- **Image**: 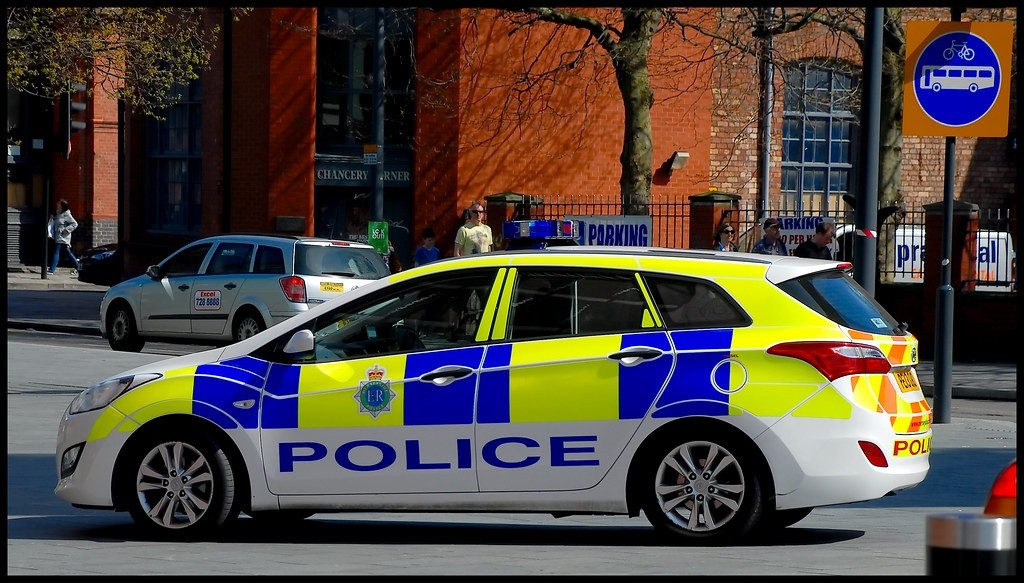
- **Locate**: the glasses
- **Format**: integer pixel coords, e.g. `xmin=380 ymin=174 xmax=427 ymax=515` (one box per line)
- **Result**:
xmin=722 ymin=230 xmax=735 ymax=234
xmin=471 ymin=210 xmax=485 ymax=214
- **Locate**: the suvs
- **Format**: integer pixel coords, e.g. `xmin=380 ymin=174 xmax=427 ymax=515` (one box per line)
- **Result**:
xmin=98 ymin=233 xmax=390 ymax=353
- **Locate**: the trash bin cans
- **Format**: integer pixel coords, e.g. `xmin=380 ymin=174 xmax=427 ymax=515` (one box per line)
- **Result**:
xmin=925 ymin=511 xmax=1016 ymax=576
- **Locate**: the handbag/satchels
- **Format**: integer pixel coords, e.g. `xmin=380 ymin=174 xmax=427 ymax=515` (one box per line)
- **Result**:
xmin=48 ymin=219 xmax=55 ymax=239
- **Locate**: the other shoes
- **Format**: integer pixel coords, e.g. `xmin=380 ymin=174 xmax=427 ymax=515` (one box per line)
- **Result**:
xmin=71 ymin=269 xmax=78 ymax=275
xmin=47 ymin=271 xmax=53 ymax=275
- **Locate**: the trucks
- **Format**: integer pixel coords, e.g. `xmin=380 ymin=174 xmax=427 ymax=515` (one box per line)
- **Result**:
xmin=827 ymin=221 xmax=1016 ymax=293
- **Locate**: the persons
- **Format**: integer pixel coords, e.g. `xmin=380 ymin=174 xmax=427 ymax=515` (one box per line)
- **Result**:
xmin=710 ymin=221 xmax=739 ymax=254
xmin=45 ymin=199 xmax=80 ymax=273
xmin=452 ymin=203 xmax=494 ymax=258
xmin=793 ymin=219 xmax=837 ymax=259
xmin=751 ymin=217 xmax=788 ymax=257
xmin=413 ymin=228 xmax=440 ymax=266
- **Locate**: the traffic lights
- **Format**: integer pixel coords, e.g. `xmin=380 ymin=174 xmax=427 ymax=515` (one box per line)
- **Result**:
xmin=54 ymin=72 xmax=86 ymax=158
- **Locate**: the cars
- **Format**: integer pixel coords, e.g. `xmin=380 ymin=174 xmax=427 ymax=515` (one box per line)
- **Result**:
xmin=78 ymin=242 xmax=118 ymax=287
xmin=50 ymin=243 xmax=932 ymax=550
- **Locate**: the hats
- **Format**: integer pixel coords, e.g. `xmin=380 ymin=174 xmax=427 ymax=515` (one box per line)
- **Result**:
xmin=763 ymin=218 xmax=779 ymax=230
